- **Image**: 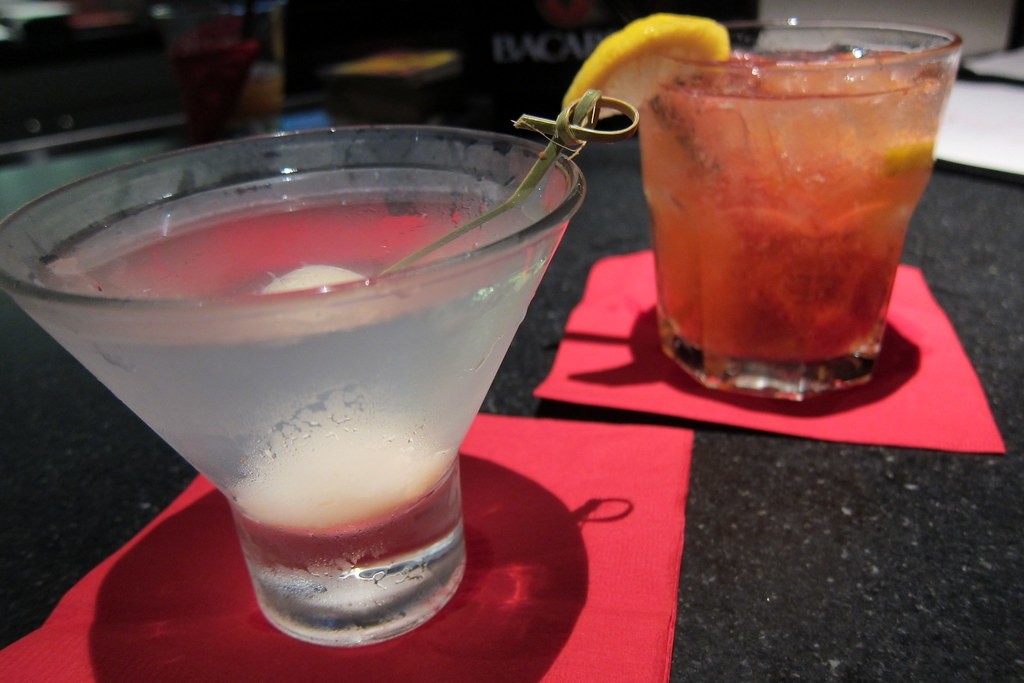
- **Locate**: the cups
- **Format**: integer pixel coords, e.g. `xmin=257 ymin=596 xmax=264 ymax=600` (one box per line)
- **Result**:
xmin=0 ymin=125 xmax=587 ymax=647
xmin=637 ymin=16 xmax=963 ymax=400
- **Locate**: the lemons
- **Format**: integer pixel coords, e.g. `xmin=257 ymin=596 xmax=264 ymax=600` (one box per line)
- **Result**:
xmin=560 ymin=14 xmax=730 ymax=120
